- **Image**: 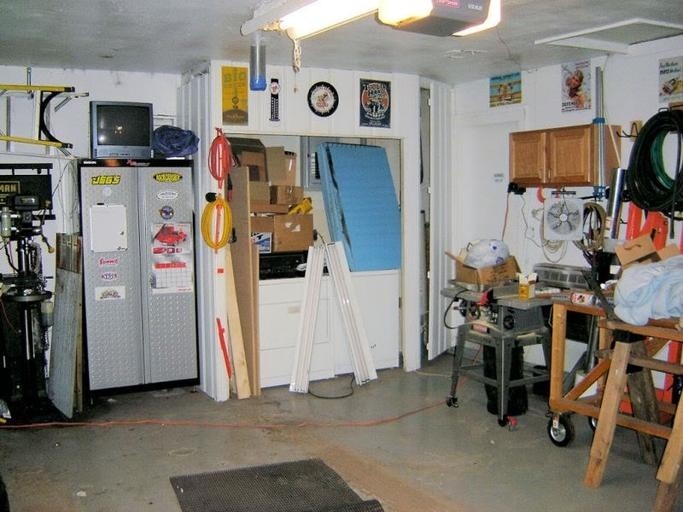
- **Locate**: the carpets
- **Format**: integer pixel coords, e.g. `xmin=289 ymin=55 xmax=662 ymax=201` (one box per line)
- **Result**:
xmin=167 ymin=455 xmax=385 ymax=512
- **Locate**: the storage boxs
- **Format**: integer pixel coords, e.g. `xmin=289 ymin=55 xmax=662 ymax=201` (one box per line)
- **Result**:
xmin=443 ymin=250 xmax=523 ymax=291
xmin=238 ymin=146 xmax=314 ymax=252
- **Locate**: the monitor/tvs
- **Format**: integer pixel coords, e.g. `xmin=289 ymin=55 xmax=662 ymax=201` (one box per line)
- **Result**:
xmin=89 ymin=101 xmax=153 ymax=160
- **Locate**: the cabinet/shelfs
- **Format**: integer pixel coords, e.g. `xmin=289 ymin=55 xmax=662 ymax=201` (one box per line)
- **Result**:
xmin=508 ymin=123 xmax=623 ymax=189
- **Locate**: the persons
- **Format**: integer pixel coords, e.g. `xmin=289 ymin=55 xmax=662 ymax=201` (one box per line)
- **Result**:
xmin=560 ymin=70 xmax=585 ymax=110
